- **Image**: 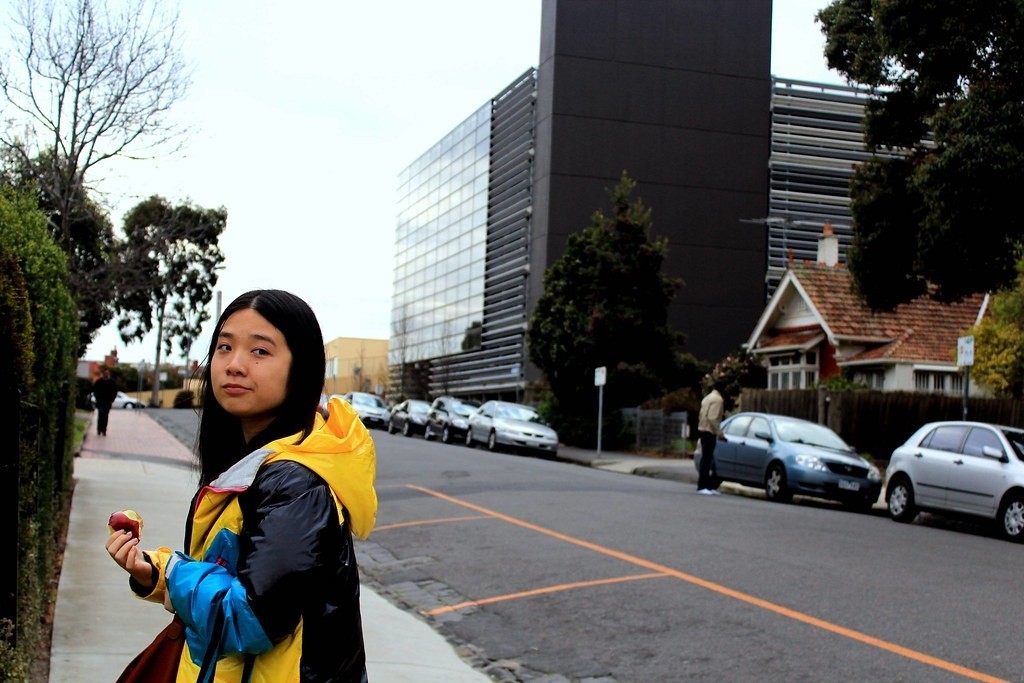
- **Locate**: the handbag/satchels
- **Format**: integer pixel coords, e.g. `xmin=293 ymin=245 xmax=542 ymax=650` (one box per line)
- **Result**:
xmin=116 ymin=613 xmax=186 ymax=683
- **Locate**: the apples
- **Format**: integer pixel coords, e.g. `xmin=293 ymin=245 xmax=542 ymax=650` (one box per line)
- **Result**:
xmin=108 ymin=509 xmax=143 ymax=544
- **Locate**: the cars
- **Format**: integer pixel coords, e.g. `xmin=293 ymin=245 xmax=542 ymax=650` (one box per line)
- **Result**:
xmin=87 ymin=390 xmax=147 ymax=411
xmin=467 ymin=400 xmax=559 ymax=459
xmin=386 ymin=399 xmax=428 ymax=437
xmin=884 ymin=422 xmax=1024 ymax=544
xmin=344 ymin=392 xmax=391 ymax=430
xmin=316 ymin=393 xmax=347 ymax=419
xmin=426 ymin=395 xmax=484 ymax=444
xmin=693 ymin=411 xmax=883 ymax=511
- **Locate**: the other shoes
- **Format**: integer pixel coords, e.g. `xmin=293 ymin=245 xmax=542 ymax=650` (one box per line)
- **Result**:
xmin=697 ymin=489 xmax=712 ymax=495
xmin=710 ymin=489 xmax=721 ymax=496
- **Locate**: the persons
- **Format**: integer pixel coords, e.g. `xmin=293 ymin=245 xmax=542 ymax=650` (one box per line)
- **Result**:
xmin=696 ymin=379 xmax=727 ymax=495
xmin=105 ymin=290 xmax=379 ymax=683
xmin=92 ymin=370 xmax=117 ymax=436
